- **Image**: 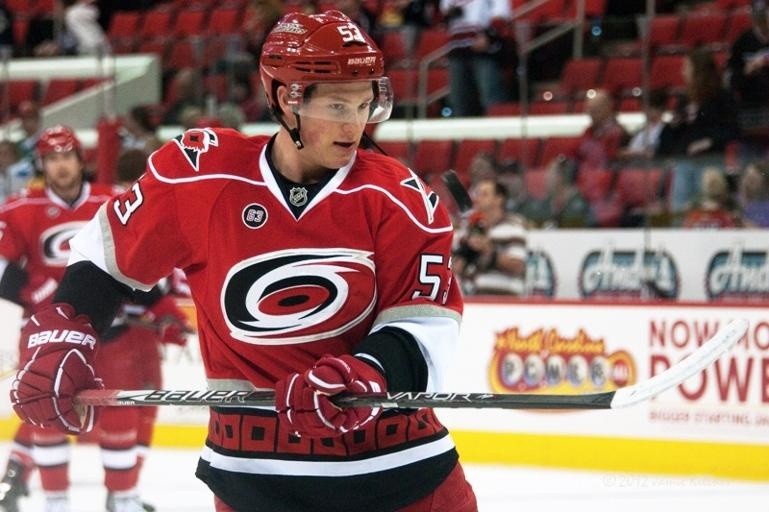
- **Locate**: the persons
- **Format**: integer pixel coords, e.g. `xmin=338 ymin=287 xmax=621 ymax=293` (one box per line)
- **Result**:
xmin=0 ymin=123 xmax=147 ymax=511
xmin=0 ymin=150 xmax=194 ymax=510
xmin=0 ymin=1 xmax=769 ymax=297
xmin=11 ymin=8 xmax=480 ymax=512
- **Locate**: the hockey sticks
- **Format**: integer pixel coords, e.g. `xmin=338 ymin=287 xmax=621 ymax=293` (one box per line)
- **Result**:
xmin=69 ymin=308 xmax=751 ymax=409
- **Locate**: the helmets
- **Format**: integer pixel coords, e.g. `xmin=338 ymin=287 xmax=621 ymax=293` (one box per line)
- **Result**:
xmin=257 ymin=8 xmax=389 ymax=113
xmin=35 ymin=125 xmax=82 ymax=160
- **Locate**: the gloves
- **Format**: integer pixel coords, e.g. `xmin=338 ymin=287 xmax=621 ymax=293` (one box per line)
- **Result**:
xmin=145 ymin=294 xmax=194 ymax=349
xmin=7 ymin=301 xmax=107 ymax=437
xmin=274 ymin=351 xmax=393 ymax=441
xmin=17 ymin=270 xmax=64 ymax=314
